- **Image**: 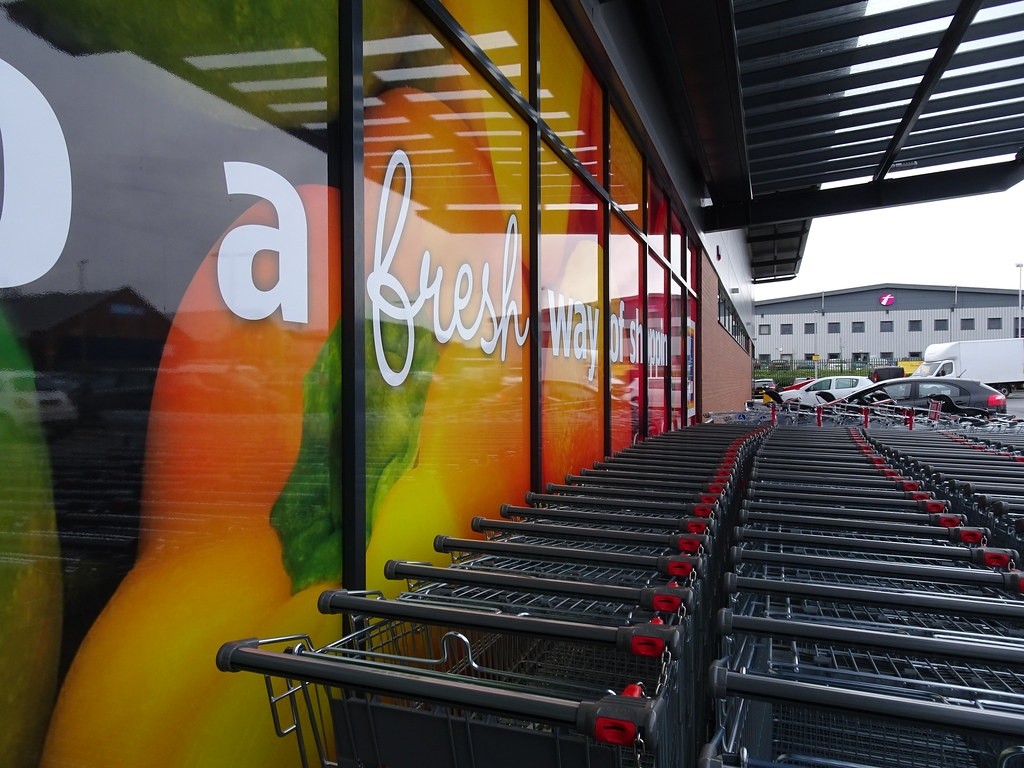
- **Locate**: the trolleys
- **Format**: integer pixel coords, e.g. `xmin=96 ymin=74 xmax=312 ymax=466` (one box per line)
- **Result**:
xmin=217 ymin=410 xmax=1024 ymax=768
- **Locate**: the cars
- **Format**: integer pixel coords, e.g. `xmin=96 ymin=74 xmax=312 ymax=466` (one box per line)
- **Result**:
xmin=0 ymin=344 xmax=698 ymax=768
xmin=813 ymin=376 xmax=1008 ymax=418
xmin=749 ymin=364 xmax=906 ymax=404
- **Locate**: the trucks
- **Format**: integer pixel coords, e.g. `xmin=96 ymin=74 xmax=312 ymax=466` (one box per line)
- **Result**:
xmin=913 ymin=337 xmax=1023 ymax=396
